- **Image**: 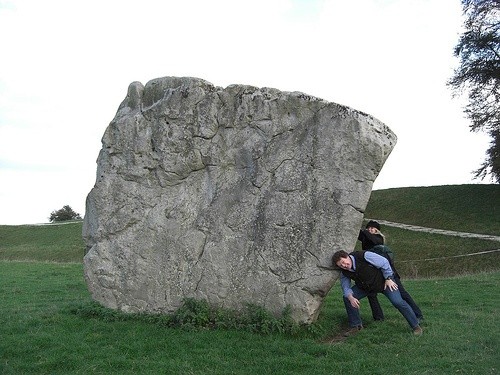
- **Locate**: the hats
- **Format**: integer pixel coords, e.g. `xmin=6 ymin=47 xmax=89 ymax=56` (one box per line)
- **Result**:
xmin=366 ymin=220 xmax=381 ymax=230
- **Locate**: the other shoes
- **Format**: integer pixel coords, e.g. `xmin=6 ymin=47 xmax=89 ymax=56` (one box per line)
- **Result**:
xmin=344 ymin=327 xmax=360 ymax=337
xmin=413 ymin=325 xmax=422 ymax=336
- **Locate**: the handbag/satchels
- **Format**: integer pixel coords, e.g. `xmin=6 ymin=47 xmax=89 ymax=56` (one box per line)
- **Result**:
xmin=368 ymin=245 xmax=394 ymax=265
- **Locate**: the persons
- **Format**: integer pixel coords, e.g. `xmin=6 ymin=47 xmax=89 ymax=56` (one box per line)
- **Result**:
xmin=332 ymin=250 xmax=422 ymax=336
xmin=355 ymin=221 xmax=423 ymax=322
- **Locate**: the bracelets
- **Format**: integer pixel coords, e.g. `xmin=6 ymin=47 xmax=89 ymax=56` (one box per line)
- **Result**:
xmin=385 ymin=276 xmax=391 ymax=280
